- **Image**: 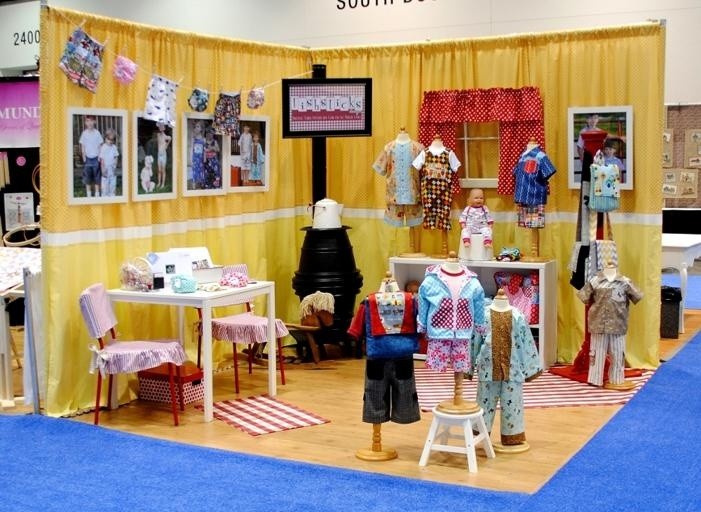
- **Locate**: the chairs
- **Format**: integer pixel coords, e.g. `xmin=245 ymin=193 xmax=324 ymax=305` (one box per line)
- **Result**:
xmin=77 ymin=283 xmax=188 ymax=427
xmin=194 ymin=264 xmax=286 ymax=395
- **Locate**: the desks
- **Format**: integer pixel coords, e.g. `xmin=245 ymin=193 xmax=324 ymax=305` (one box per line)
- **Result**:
xmin=100 ymin=274 xmax=278 ymax=426
xmin=659 ymin=233 xmax=701 ymax=335
xmin=1 ymin=246 xmax=44 ymax=411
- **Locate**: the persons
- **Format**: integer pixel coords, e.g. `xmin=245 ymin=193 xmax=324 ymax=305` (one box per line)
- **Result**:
xmin=347 ymin=271 xmax=422 ymax=423
xmin=577 ymin=115 xmax=606 ymax=183
xmin=463 ymin=289 xmax=543 ymax=443
xmin=204 ymin=129 xmax=220 ymax=188
xmin=237 ymin=125 xmax=253 ymax=184
xmin=577 ymin=268 xmax=642 ymax=388
xmin=99 ymin=128 xmax=120 ymax=196
xmin=374 ymin=128 xmax=426 ymax=229
xmin=514 ymin=137 xmax=557 ymax=229
xmin=251 ymin=132 xmax=264 ymax=185
xmin=156 ymin=125 xmax=171 ymax=191
xmin=412 ymin=136 xmax=463 ymax=232
xmin=191 ymin=121 xmax=207 ymax=189
xmin=417 ymin=251 xmax=486 ymax=374
xmin=79 ymin=115 xmax=105 ymax=197
xmin=459 ymin=188 xmax=493 ymax=247
xmin=604 ymin=141 xmax=624 ymax=183
xmin=140 ymin=155 xmax=155 ymax=193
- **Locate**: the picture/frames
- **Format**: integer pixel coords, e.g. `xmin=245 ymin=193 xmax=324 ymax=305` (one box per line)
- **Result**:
xmin=65 ymin=106 xmax=128 ymax=205
xmin=180 ymin=110 xmax=227 ymax=197
xmin=132 ymin=109 xmax=178 ymax=203
xmin=227 ymin=113 xmax=270 ymax=193
xmin=565 ymin=106 xmax=634 ymax=191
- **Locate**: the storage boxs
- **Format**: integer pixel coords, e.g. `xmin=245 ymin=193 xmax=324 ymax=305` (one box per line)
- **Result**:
xmin=134 ymin=365 xmax=205 ymax=408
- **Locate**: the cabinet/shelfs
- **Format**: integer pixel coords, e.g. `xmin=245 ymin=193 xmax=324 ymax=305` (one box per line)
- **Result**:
xmin=387 ymin=256 xmax=559 ymax=371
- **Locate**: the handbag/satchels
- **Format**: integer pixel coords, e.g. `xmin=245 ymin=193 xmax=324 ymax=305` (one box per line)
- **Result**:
xmin=588 ymin=149 xmax=620 ymax=212
xmin=584 ymin=239 xmax=618 ymax=283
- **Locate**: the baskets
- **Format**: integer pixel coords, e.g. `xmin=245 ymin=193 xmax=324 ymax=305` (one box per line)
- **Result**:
xmin=137 ymin=370 xmax=204 ymax=407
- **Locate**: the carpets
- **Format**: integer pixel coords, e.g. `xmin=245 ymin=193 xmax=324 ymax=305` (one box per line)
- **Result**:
xmin=410 ymin=366 xmax=658 ymax=412
xmin=193 ymin=393 xmax=330 ymax=437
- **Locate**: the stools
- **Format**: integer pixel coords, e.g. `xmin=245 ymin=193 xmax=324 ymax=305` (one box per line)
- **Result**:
xmin=418 ymin=406 xmax=496 ymax=473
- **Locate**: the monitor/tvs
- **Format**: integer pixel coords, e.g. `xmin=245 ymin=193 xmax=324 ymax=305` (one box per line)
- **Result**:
xmin=281 ymin=77 xmax=373 ymax=139
xmin=1 ymin=76 xmax=40 ymax=147
xmin=662 ymin=207 xmax=701 ymax=235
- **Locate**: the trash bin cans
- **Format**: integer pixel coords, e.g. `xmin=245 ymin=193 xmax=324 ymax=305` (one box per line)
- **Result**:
xmin=660 ymin=286 xmax=682 ymax=339
